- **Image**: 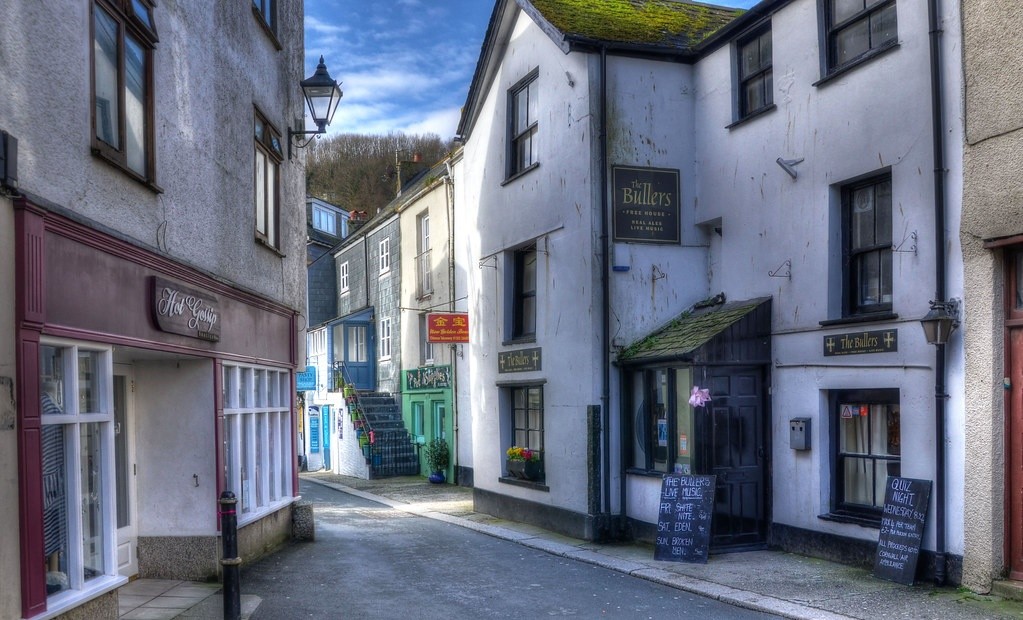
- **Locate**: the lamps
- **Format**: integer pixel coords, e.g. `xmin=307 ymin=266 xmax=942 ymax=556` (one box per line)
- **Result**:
xmin=287 ymin=53 xmax=343 ymax=160
xmin=922 ymin=298 xmax=960 ymax=345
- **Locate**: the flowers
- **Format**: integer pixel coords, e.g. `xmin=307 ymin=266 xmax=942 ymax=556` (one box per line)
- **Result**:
xmin=509 ymin=447 xmax=534 ymax=461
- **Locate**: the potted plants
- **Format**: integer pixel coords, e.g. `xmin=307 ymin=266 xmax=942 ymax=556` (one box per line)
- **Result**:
xmin=336 ymin=373 xmax=373 ymax=459
xmin=424 ymin=438 xmax=449 ymax=485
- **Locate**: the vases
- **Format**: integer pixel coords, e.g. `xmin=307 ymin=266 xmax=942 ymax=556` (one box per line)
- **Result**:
xmin=507 ymin=458 xmax=541 ymax=481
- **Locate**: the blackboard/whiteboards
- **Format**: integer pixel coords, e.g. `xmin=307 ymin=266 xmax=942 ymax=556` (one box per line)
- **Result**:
xmin=653 ymin=473 xmax=717 ymax=565
xmin=872 ymin=475 xmax=933 ymax=587
